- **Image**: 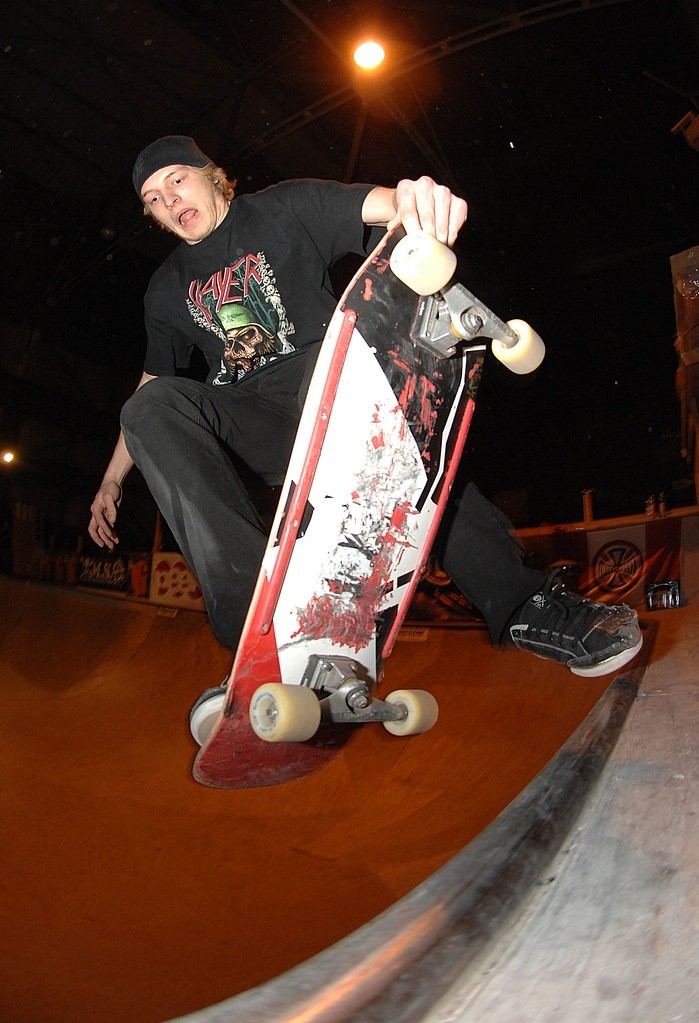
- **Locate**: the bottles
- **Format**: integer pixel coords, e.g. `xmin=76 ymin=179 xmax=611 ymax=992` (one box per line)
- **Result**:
xmin=582 ymin=489 xmax=593 ymax=521
xmin=658 ymin=492 xmax=666 ymax=518
xmin=645 ymin=495 xmax=656 ymax=521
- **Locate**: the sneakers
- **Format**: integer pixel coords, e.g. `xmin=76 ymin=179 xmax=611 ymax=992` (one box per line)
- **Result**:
xmin=190 ymin=674 xmax=229 ymax=747
xmin=506 ymin=575 xmax=643 ymax=677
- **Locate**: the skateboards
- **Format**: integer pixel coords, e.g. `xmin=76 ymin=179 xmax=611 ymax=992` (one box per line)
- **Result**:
xmin=187 ymin=217 xmax=549 ymax=794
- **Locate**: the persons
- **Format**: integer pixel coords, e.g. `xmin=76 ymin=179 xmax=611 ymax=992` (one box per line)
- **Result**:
xmin=89 ymin=138 xmax=641 ymax=672
xmin=76 ymin=528 xmax=108 ymax=555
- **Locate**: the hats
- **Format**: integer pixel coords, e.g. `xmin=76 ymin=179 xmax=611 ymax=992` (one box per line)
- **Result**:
xmin=130 ymin=135 xmax=217 ymax=200
